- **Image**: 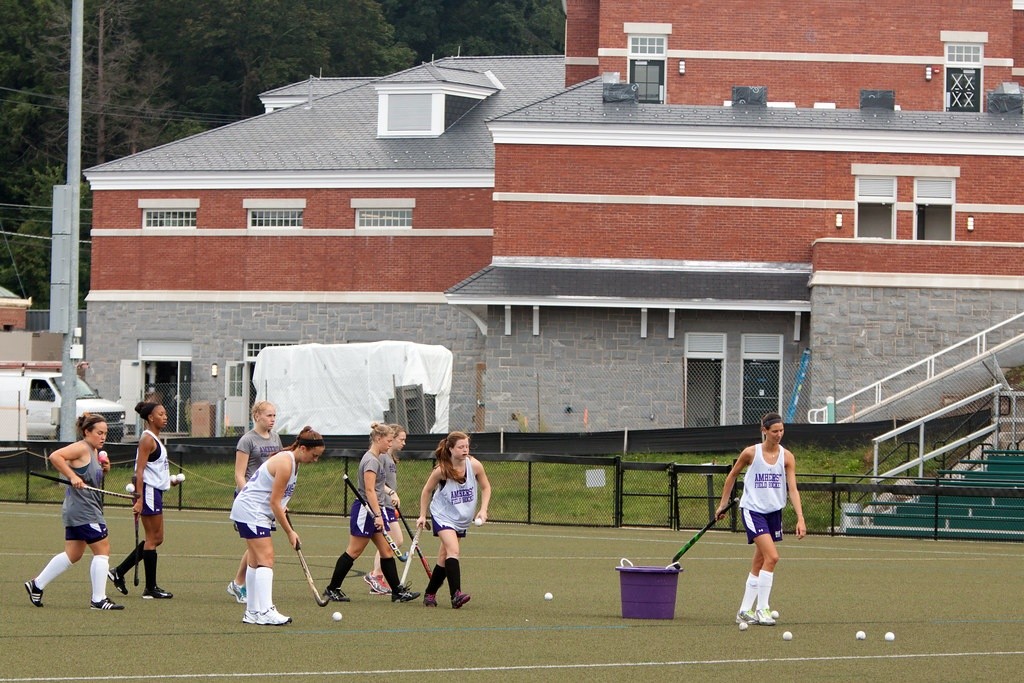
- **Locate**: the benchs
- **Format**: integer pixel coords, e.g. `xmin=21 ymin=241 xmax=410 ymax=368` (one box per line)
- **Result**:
xmin=845 ymin=450 xmax=1024 ymax=539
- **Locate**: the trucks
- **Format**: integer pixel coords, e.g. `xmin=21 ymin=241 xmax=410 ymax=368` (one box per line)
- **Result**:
xmin=0 ymin=359 xmax=127 ymax=443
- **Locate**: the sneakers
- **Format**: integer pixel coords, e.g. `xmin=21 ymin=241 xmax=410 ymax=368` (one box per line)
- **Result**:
xmin=324 ymin=586 xmax=350 ymax=603
xmin=242 ymin=610 xmax=258 ymax=623
xmin=451 ymin=589 xmax=471 ymax=608
xmin=90 ymin=597 xmax=124 ymax=611
xmin=227 ymin=581 xmax=246 ymax=603
xmin=392 ymin=585 xmax=422 ymax=602
xmin=736 ymin=608 xmax=760 ymax=625
xmin=109 ymin=567 xmax=129 ymax=596
xmin=364 ymin=572 xmax=388 ymax=594
xmin=24 ymin=580 xmax=44 ymax=608
xmin=142 ymin=586 xmax=173 ymax=600
xmin=255 ymin=607 xmax=293 ymax=626
xmin=369 ymin=583 xmax=393 ymax=595
xmin=423 ymin=594 xmax=437 ymax=607
xmin=756 ymin=607 xmax=776 ymax=625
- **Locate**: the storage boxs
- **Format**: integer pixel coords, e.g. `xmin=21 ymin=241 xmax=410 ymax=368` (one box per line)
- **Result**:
xmin=192 ymin=405 xmax=216 ymax=437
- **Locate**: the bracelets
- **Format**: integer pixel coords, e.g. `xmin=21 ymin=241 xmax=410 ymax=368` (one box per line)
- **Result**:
xmin=797 ymin=513 xmax=803 ymax=515
xmin=374 ymin=512 xmax=382 ymax=517
xmin=388 ymin=489 xmax=395 ymax=495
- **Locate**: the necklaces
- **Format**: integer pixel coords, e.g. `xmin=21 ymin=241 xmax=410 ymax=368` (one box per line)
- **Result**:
xmin=763 ymin=442 xmax=779 ymax=458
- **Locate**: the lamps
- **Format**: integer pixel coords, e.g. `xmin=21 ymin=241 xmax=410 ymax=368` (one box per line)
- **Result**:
xmin=967 ymin=215 xmax=974 ymax=233
xmin=678 ymin=59 xmax=686 ymax=76
xmin=212 ymin=362 xmax=218 ymax=377
xmin=926 ymin=65 xmax=932 ymax=82
xmin=835 ymin=212 xmax=842 ymax=229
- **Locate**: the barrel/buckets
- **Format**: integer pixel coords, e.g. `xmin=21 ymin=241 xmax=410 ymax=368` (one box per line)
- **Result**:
xmin=615 ymin=558 xmax=684 ymax=620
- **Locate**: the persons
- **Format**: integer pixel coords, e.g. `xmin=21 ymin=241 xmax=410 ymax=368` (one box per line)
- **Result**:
xmin=38 ymin=383 xmax=52 ymax=400
xmin=227 ymin=401 xmax=284 ymax=603
xmin=25 ymin=413 xmax=125 ymax=611
xmin=325 ymin=423 xmax=421 ymax=603
xmin=108 ymin=401 xmax=179 ymax=598
xmin=163 ymin=375 xmax=177 ymax=432
xmin=230 ymin=425 xmax=325 ymax=625
xmin=184 ymin=397 xmax=190 ymax=431
xmin=416 ymin=432 xmax=492 ymax=608
xmin=716 ymin=413 xmax=806 ymax=626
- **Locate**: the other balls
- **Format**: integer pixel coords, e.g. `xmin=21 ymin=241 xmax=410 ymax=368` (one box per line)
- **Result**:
xmin=771 ymin=611 xmax=779 ymax=618
xmin=545 ymin=593 xmax=553 ymax=600
xmin=783 ymin=631 xmax=792 ymax=640
xmin=885 ymin=632 xmax=895 ymax=641
xmin=126 ymin=483 xmax=135 ymax=493
xmin=475 ymin=518 xmax=482 ymax=526
xmin=170 ymin=473 xmax=186 ymax=483
xmin=856 ymin=631 xmax=866 ymax=640
xmin=739 ymin=622 xmax=748 ymax=630
xmin=332 ymin=612 xmax=342 ymax=621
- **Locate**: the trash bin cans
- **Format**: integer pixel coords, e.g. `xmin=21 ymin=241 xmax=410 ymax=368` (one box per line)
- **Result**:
xmin=839 ymin=503 xmax=863 ymax=532
xmin=191 ymin=401 xmax=215 ymax=438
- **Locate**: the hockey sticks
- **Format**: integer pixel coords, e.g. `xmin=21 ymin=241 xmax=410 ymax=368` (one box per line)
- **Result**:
xmin=131 ymin=475 xmax=140 ymax=587
xmin=671 ymin=497 xmax=740 ymax=572
xmin=29 ymin=470 xmax=141 ymax=499
xmin=342 ymin=473 xmax=409 ymax=562
xmin=398 ymin=520 xmax=431 ymax=591
xmin=285 ymin=510 xmax=331 ymax=608
xmin=395 ymin=504 xmax=432 ymax=579
